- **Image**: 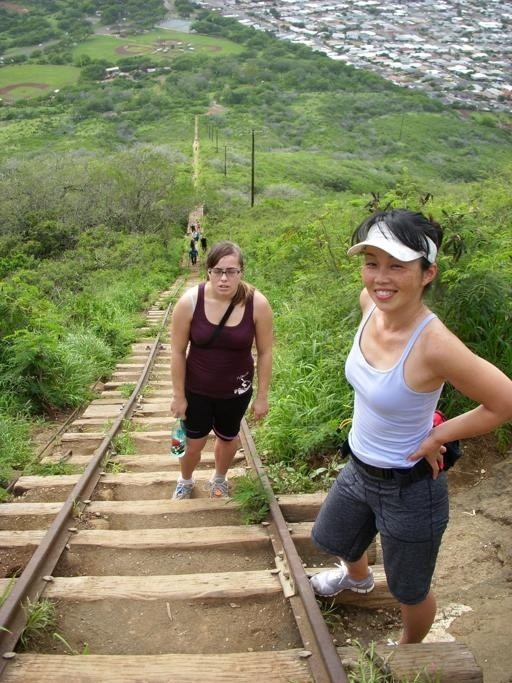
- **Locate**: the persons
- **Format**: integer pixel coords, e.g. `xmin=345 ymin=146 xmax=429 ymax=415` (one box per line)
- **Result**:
xmin=309 ymin=208 xmax=512 ymax=646
xmin=170 ymin=241 xmax=273 ymax=500
xmin=189 ymin=219 xmax=207 ymax=265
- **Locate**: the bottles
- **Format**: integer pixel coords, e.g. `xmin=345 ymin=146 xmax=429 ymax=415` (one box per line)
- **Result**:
xmin=170 ymin=417 xmax=186 ymax=458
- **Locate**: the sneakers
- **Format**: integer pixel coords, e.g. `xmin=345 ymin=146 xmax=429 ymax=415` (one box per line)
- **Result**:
xmin=172 ymin=476 xmax=195 ymax=499
xmin=210 ymin=473 xmax=228 ymax=498
xmin=310 ymin=567 xmax=374 ymax=597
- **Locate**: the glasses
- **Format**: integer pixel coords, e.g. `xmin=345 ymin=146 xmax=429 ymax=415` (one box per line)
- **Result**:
xmin=208 ymin=267 xmax=243 ymax=274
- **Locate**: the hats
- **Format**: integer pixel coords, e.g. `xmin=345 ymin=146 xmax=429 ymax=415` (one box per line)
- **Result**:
xmin=346 ymin=221 xmax=438 ymax=265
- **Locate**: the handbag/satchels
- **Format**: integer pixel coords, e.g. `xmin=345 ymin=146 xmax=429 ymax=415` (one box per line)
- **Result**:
xmin=433 ymin=410 xmax=461 ymax=472
